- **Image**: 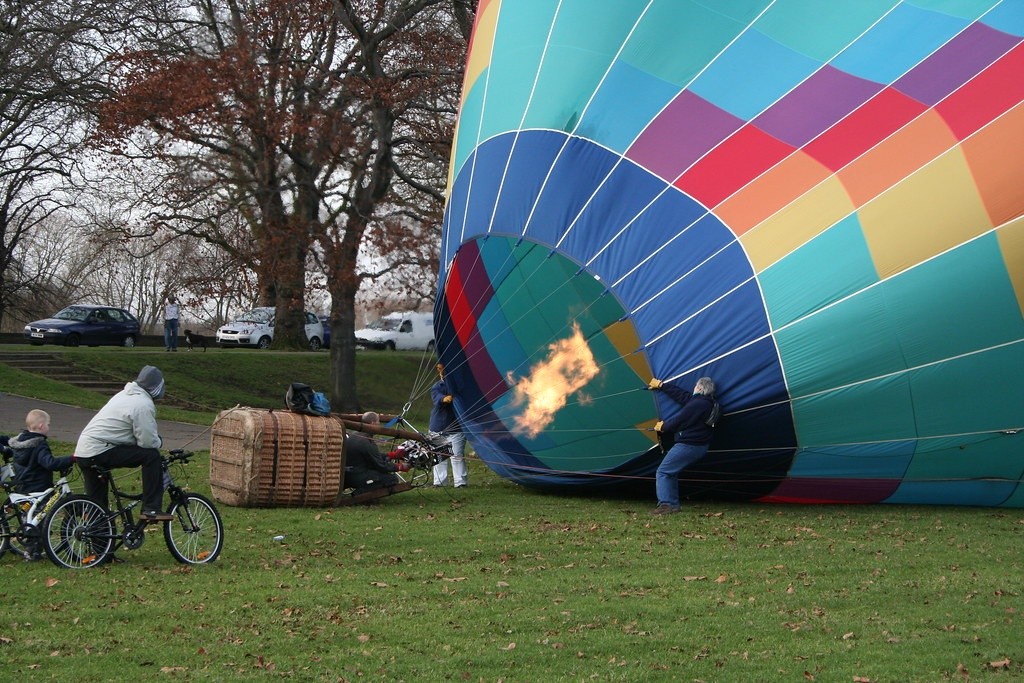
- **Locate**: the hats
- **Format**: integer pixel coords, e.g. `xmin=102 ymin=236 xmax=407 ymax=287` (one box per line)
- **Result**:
xmin=436 ymin=363 xmax=445 ymax=370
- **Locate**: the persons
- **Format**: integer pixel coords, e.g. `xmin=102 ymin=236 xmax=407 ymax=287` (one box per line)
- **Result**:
xmin=74 ymin=365 xmax=174 ymax=566
xmin=161 ymin=294 xmax=180 ymax=352
xmin=647 ymin=377 xmax=720 ymax=515
xmin=428 ymin=363 xmax=470 ymax=488
xmin=7 ymin=409 xmax=77 ymax=561
xmin=343 ymin=411 xmax=411 ymax=506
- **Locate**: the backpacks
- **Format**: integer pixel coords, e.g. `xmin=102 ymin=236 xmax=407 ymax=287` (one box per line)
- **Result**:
xmin=699 ymin=394 xmax=721 ymax=428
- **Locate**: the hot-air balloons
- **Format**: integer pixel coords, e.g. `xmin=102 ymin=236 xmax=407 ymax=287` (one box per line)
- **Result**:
xmin=210 ymin=0 xmax=1024 ymax=517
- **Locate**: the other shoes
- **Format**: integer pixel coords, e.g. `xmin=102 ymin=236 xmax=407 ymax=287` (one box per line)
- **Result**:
xmin=672 ymin=507 xmax=682 ymax=513
xmin=430 ymin=484 xmax=446 ymax=489
xmin=171 ymin=347 xmax=177 ymax=352
xmin=32 ymin=553 xmax=44 ymax=561
xmin=166 ymin=349 xmax=170 ymax=352
xmin=458 ymin=485 xmax=467 ymax=489
xmin=23 ymin=551 xmax=32 ymax=561
xmin=655 ymin=505 xmax=672 ymax=515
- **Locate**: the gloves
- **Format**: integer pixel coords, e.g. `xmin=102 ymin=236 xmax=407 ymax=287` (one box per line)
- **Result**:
xmin=387 ymin=448 xmax=406 ymax=459
xmin=654 ymin=420 xmax=664 ymax=432
xmin=443 ymin=395 xmax=452 ymax=403
xmin=394 ymin=462 xmax=412 ymax=472
xmin=648 ymin=378 xmax=662 ymax=390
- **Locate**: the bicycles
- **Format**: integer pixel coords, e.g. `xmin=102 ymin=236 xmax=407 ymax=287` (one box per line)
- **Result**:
xmin=43 ymin=451 xmax=225 ymax=570
xmin=0 ymin=449 xmax=98 ymax=565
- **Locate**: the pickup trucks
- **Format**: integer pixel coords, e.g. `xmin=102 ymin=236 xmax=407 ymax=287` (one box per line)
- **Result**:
xmin=354 ymin=311 xmax=436 ymax=352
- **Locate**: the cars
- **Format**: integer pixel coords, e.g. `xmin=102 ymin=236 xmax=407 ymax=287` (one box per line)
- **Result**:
xmin=24 ymin=305 xmax=140 ymax=348
xmin=216 ymin=307 xmax=324 ymax=351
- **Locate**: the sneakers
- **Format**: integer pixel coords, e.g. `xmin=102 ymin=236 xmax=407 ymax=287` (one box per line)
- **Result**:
xmin=140 ymin=509 xmax=173 ymax=521
